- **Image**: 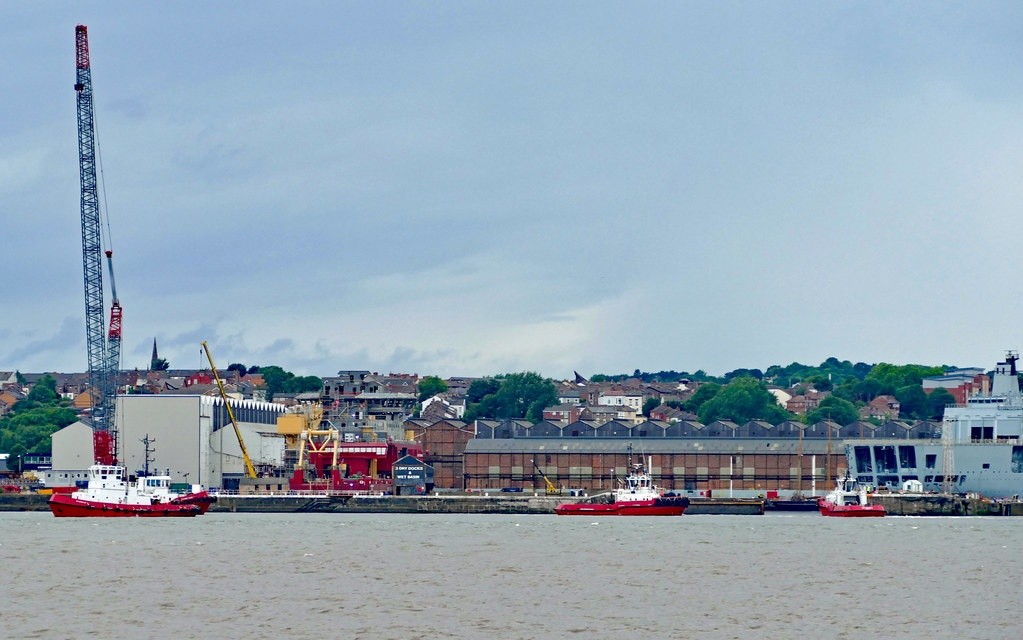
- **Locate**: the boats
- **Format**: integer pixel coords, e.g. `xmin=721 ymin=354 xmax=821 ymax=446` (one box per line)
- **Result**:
xmin=46 ymin=463 xmax=218 ymax=518
xmin=553 ymin=455 xmax=690 ymax=516
xmin=817 ymin=470 xmax=888 ymax=517
xmin=841 ymin=348 xmax=1023 ymax=504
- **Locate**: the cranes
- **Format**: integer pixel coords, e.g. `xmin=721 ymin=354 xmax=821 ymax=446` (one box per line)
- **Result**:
xmin=71 ymin=24 xmax=125 ymax=468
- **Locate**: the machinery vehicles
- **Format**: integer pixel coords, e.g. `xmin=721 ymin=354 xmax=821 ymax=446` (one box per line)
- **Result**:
xmin=199 ymin=341 xmax=276 ymax=478
xmin=530 ymin=458 xmax=557 ymax=495
